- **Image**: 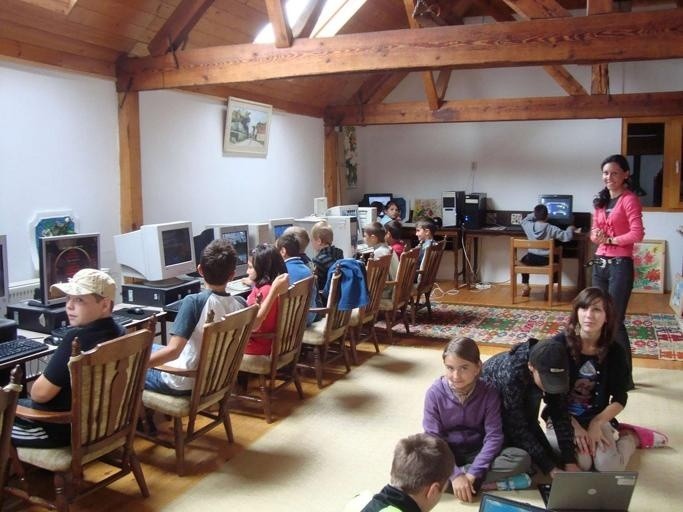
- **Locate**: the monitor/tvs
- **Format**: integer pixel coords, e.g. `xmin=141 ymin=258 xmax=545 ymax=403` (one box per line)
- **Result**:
xmin=38 ymin=233 xmax=100 ymax=306
xmin=368 ymin=194 xmax=389 ymax=216
xmin=197 ymin=224 xmax=249 ymax=280
xmin=540 ymin=195 xmax=572 ymax=225
xmin=115 ymin=222 xmax=196 ymax=287
xmin=271 ymin=217 xmax=298 ymax=244
xmin=335 ymin=204 xmax=358 ymax=216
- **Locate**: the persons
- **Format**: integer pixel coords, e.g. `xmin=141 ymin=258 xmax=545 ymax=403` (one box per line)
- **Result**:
xmin=346 ymin=428 xmax=456 ymax=512
xmin=419 ymin=335 xmax=534 ymax=504
xmin=133 ymin=238 xmax=250 ymax=436
xmin=481 ymin=335 xmax=584 ymax=481
xmin=518 ymin=202 xmax=577 ymax=302
xmin=583 ymin=152 xmax=646 ymax=393
xmin=238 ymin=197 xmax=441 ymax=357
xmin=0 ymin=266 xmax=133 ymax=451
xmin=548 ymin=287 xmax=670 ymax=474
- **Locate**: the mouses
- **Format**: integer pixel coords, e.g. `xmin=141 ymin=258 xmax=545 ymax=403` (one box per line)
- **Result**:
xmin=46 ymin=333 xmax=66 ymax=346
xmin=129 ymin=306 xmax=145 ymax=315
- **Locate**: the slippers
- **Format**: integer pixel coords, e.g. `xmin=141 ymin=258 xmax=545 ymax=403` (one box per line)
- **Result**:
xmin=617 ymin=423 xmax=669 ymax=449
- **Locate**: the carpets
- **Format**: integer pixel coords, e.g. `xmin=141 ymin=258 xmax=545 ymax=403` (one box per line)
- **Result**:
xmin=157 ymin=345 xmax=683 ymax=512
xmin=354 ymin=300 xmax=683 ymax=362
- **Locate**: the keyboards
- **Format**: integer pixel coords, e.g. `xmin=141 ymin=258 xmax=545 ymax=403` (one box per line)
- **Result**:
xmin=1 ymin=337 xmax=48 ymax=362
xmin=54 ymin=316 xmax=131 ymax=337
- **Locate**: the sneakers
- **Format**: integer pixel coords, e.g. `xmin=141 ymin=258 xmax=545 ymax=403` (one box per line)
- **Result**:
xmin=495 ymin=472 xmax=533 ymax=490
xmin=544 ymin=284 xmax=549 ymax=301
xmin=521 ymin=283 xmax=531 ymax=297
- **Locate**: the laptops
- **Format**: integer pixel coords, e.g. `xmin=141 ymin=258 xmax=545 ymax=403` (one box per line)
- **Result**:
xmin=538 ymin=471 xmax=638 ymax=511
xmin=479 ymin=491 xmax=540 ymax=511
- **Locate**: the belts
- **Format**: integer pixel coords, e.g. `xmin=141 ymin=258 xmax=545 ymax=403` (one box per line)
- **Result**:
xmin=583 ymin=256 xmax=634 ymax=268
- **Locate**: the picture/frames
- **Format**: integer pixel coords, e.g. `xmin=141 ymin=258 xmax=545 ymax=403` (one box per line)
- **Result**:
xmin=668 ymin=273 xmax=683 ymax=318
xmin=222 ymin=96 xmax=273 ymax=158
xmin=632 ymin=238 xmax=665 ymax=294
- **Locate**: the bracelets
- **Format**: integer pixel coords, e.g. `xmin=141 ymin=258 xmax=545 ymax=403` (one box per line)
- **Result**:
xmin=608 ymin=237 xmax=612 ymax=245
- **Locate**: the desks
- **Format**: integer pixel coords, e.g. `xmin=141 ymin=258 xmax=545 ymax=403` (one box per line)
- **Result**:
xmin=359 ymin=226 xmax=587 ymax=294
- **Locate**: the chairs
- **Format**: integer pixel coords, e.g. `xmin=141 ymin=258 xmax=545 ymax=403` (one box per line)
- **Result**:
xmin=510 ymin=235 xmax=563 ymax=307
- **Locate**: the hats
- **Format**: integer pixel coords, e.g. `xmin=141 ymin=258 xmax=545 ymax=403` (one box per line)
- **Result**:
xmin=528 ymin=338 xmax=570 ymax=394
xmin=49 ymin=268 xmax=117 ymax=302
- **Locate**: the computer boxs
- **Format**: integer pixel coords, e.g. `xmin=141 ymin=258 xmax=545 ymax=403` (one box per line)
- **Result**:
xmin=464 ymin=194 xmax=487 ymax=231
xmin=327 ymin=215 xmax=357 ymax=257
xmin=442 ymin=191 xmax=463 ymax=231
xmin=250 ymin=224 xmax=271 ymax=248
xmin=359 ymin=208 xmax=376 ymax=243
xmin=5 ymin=298 xmax=70 ymax=335
xmin=121 ymin=280 xmax=201 ymax=305
